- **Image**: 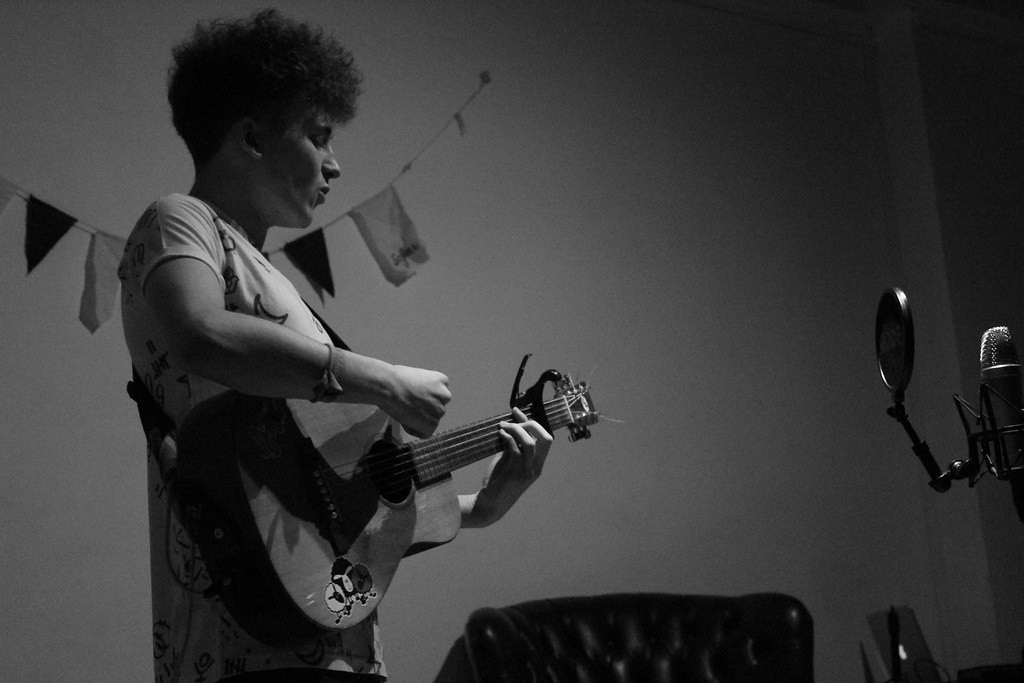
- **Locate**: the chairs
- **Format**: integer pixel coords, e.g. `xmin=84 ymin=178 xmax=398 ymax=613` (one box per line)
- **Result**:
xmin=464 ymin=591 xmax=815 ymax=683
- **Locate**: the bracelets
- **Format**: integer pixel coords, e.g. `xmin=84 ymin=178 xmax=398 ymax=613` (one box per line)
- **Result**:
xmin=309 ymin=343 xmax=346 ymax=404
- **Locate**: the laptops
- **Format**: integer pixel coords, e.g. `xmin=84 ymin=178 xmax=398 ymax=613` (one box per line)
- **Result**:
xmin=867 ymin=605 xmax=943 ymax=683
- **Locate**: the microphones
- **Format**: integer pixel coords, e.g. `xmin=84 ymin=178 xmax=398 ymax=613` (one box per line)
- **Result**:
xmin=979 ymin=326 xmax=1024 ymax=522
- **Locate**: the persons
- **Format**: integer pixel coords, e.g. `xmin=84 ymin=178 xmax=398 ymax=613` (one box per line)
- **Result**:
xmin=116 ymin=7 xmax=553 ymax=683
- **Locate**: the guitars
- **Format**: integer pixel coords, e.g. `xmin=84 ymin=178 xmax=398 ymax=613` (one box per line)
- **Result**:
xmin=179 ymin=351 xmax=599 ymax=642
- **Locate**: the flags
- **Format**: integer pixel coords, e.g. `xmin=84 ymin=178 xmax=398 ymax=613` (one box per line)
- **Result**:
xmin=349 ymin=186 xmax=432 ymax=286
